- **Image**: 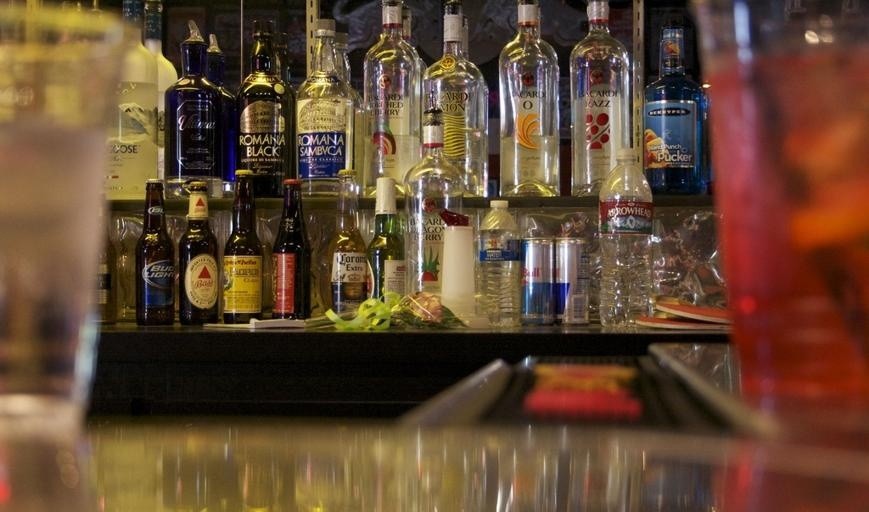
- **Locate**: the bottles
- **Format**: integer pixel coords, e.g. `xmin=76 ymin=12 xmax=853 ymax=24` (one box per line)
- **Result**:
xmin=221 ymin=169 xmax=264 ymax=323
xmin=498 ymin=0 xmax=560 ymax=196
xmin=441 ymin=226 xmax=476 ymax=322
xmin=165 ymin=18 xmax=226 ymax=175
xmin=203 ymin=33 xmax=236 ymax=175
xmin=598 ymin=147 xmax=656 ymax=327
xmin=272 ymin=31 xmax=292 ymax=85
xmin=178 ymin=180 xmax=220 ymax=324
xmin=104 ymin=0 xmax=158 ymax=198
xmin=144 ymin=0 xmax=178 ymax=181
xmin=326 ymin=170 xmax=369 ymax=313
xmin=642 ymin=20 xmax=702 ymax=190
xmin=363 ymin=0 xmax=425 ymax=185
xmin=368 ymin=176 xmax=406 ymax=306
xmin=297 ymin=19 xmax=363 ymax=195
xmin=335 ymin=32 xmax=352 ymax=86
xmin=418 ymin=0 xmax=490 ymax=201
xmin=477 ymin=200 xmax=520 ymax=327
xmin=270 ymin=179 xmax=312 ymax=320
xmin=236 ymin=19 xmax=293 ymax=181
xmin=95 ymin=191 xmax=114 ymax=328
xmin=135 ymin=179 xmax=176 ymax=326
xmin=569 ymin=0 xmax=631 ymax=197
xmin=403 ymin=107 xmax=466 ymax=302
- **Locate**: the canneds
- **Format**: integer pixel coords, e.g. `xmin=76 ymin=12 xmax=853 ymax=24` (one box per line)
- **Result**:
xmin=522 ymin=237 xmax=555 ymax=325
xmin=558 ymin=238 xmax=589 ymax=327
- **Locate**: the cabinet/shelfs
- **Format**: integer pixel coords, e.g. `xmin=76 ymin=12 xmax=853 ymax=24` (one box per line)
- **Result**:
xmin=79 ymin=0 xmax=731 ymax=437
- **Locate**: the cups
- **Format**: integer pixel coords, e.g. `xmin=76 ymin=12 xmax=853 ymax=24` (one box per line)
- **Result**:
xmin=691 ymin=0 xmax=869 ymax=448
xmin=0 ymin=7 xmax=147 ymax=449
